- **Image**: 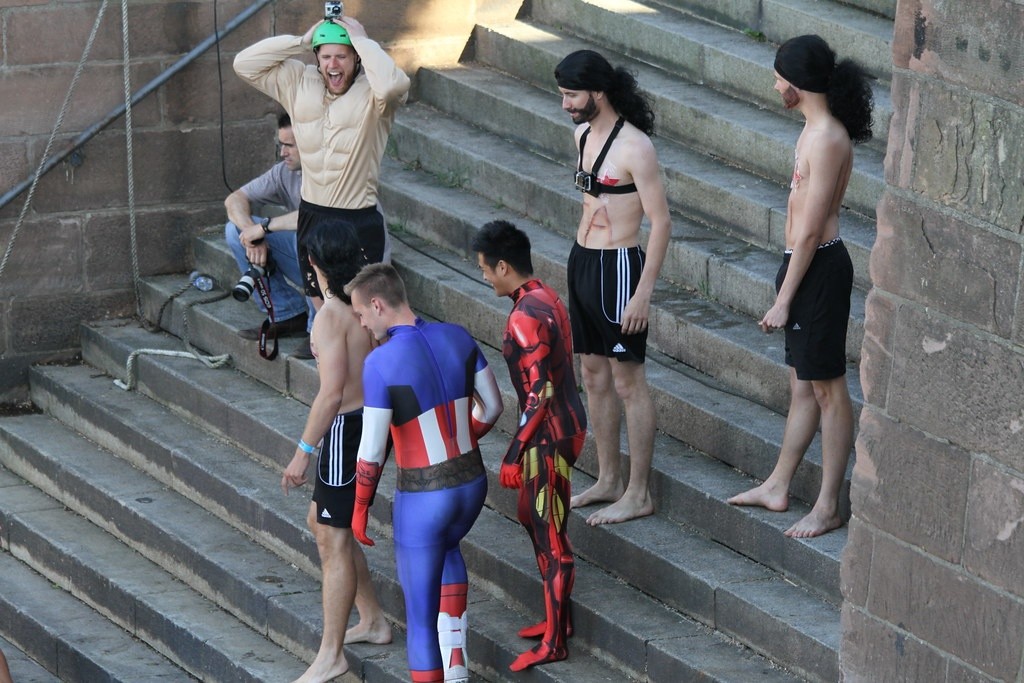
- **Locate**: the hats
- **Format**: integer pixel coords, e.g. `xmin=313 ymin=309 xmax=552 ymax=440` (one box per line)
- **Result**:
xmin=774 ymin=35 xmax=835 ymax=93
xmin=553 ymin=50 xmax=614 ymax=92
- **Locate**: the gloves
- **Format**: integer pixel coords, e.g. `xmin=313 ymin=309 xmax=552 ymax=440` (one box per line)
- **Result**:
xmin=499 ymin=462 xmax=524 ymax=490
xmin=352 ymin=507 xmax=375 ymax=547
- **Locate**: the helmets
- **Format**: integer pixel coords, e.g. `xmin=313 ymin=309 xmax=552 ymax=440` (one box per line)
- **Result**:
xmin=310 ymin=19 xmax=353 ymax=51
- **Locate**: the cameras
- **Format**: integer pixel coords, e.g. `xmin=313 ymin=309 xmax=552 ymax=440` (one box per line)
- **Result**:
xmin=325 ymin=1 xmax=343 ymax=18
xmin=231 ymin=248 xmax=277 ymax=301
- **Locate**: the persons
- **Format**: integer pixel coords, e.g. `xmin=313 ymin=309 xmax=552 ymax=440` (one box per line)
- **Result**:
xmin=472 ymin=221 xmax=588 ymax=672
xmin=232 ymin=14 xmax=409 ymax=313
xmin=726 ymin=35 xmax=876 ymax=538
xmin=282 ymin=219 xmax=390 ymax=683
xmin=556 ymin=49 xmax=672 ymax=525
xmin=224 ymin=115 xmax=392 ymax=359
xmin=343 ymin=262 xmax=504 ymax=683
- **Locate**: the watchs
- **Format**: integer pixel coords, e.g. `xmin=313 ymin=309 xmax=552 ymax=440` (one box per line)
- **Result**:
xmin=262 ymin=216 xmax=272 ymax=233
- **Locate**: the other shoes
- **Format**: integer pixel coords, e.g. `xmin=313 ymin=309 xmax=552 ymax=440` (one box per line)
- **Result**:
xmin=292 ymin=333 xmax=315 ymax=359
xmin=237 ymin=312 xmax=309 ymax=341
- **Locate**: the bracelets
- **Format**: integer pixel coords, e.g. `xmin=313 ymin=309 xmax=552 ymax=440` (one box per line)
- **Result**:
xmin=299 ymin=441 xmax=315 ymax=454
